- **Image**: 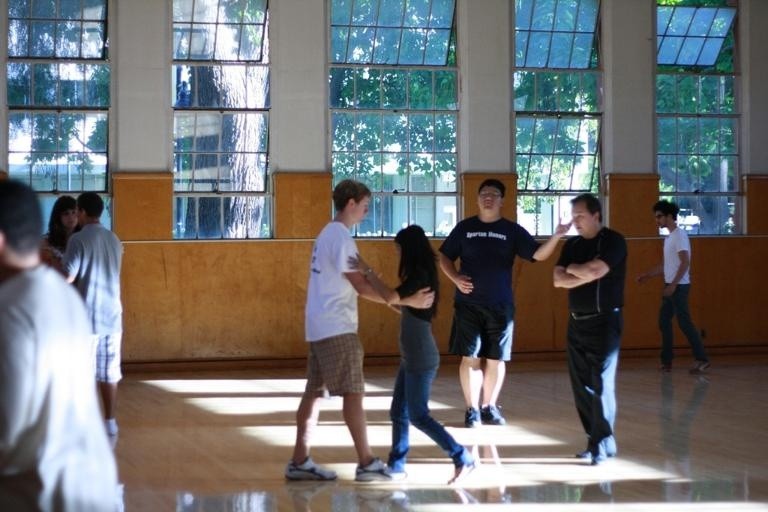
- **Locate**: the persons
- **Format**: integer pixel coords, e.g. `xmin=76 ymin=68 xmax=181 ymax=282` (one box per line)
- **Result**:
xmin=551 ymin=191 xmax=629 ymax=467
xmin=634 ymin=198 xmax=714 ymax=376
xmin=282 ymin=178 xmax=435 ymax=482
xmin=43 ymin=196 xmax=78 ymax=261
xmin=437 ymin=177 xmax=572 ymax=429
xmin=657 ymin=373 xmax=713 ymax=504
xmin=43 ymin=192 xmax=125 ymax=436
xmin=344 ymin=223 xmax=477 ymax=486
xmin=0 ymin=176 xmax=124 ymax=510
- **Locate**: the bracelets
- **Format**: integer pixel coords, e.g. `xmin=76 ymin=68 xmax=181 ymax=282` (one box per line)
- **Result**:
xmin=364 ymin=268 xmax=373 ymax=277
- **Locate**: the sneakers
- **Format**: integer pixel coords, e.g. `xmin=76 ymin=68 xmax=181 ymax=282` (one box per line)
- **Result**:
xmin=285 ymin=456 xmax=337 ymax=480
xmin=355 ymin=458 xmax=406 ymax=480
xmin=577 ymin=437 xmax=615 ymax=464
xmin=465 ymin=405 xmax=505 ymax=427
xmin=658 ymin=359 xmax=713 ymax=374
xmin=105 ymin=420 xmax=119 ymax=435
